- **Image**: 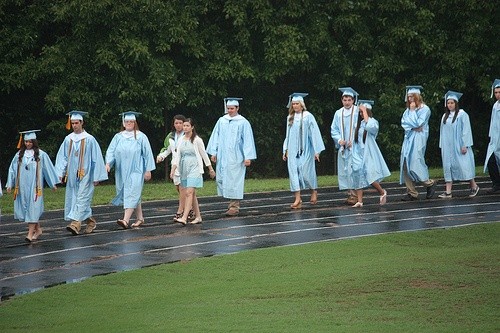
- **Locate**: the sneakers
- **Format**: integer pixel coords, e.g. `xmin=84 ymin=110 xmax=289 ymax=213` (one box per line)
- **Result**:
xmin=84 ymin=224 xmax=97 ymax=233
xmin=468 ymin=185 xmax=479 ymax=197
xmin=438 ymin=192 xmax=452 ymax=198
xmin=66 ymin=225 xmax=79 ymax=235
xmin=224 ymin=210 xmax=240 ymax=216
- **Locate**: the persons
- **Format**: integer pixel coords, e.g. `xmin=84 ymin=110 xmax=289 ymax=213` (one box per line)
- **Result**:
xmin=282 ymin=93 xmax=326 ymax=208
xmin=483 ymin=78 xmax=500 ymax=193
xmin=330 ymin=87 xmax=392 ymax=208
xmin=156 ymin=115 xmax=216 ymax=225
xmin=399 ymin=85 xmax=436 ymax=201
xmin=206 ymin=97 xmax=257 ymax=216
xmin=438 ymin=90 xmax=480 ymax=198
xmin=5 ymin=130 xmax=60 ymax=242
xmin=55 ymin=110 xmax=108 ymax=235
xmin=105 ymin=111 xmax=156 ymax=228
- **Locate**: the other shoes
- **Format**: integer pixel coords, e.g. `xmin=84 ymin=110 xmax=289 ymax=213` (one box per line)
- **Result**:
xmin=351 ymin=202 xmax=363 ymax=207
xmin=380 ymin=190 xmax=387 ymax=207
xmin=487 ymin=188 xmax=500 ymax=194
xmin=346 ymin=196 xmax=358 ymax=205
xmin=174 ymin=209 xmax=195 ymax=222
xmin=25 ymin=232 xmax=42 ymax=242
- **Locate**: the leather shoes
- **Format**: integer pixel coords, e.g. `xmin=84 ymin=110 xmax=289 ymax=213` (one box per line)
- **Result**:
xmin=426 ymin=181 xmax=437 ymax=198
xmin=401 ymin=195 xmax=419 ymax=201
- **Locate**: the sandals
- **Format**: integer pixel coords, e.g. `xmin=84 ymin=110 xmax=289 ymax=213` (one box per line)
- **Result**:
xmin=189 ymin=216 xmax=203 ymax=225
xmin=174 ymin=218 xmax=187 ymax=227
xmin=131 ymin=218 xmax=145 ymax=227
xmin=117 ymin=219 xmax=129 ymax=228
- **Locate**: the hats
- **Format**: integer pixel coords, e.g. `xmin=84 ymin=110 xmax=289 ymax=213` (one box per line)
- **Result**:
xmin=224 ymin=98 xmax=243 ymax=113
xmin=286 ymin=93 xmax=308 ymax=108
xmin=490 ymin=79 xmax=500 ymax=98
xmin=338 ymin=87 xmax=359 ymax=105
xmin=118 ymin=111 xmax=142 ymax=127
xmin=66 ymin=111 xmax=88 ymax=130
xmin=357 ymin=100 xmax=374 ymax=110
xmin=404 ymin=86 xmax=424 ymax=102
xmin=444 ymin=91 xmax=463 ymax=107
xmin=16 ymin=129 xmax=41 ymax=148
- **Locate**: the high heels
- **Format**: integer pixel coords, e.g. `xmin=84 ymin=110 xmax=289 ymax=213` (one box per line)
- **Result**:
xmin=291 ymin=198 xmax=302 ymax=208
xmin=310 ymin=189 xmax=318 ymax=204
